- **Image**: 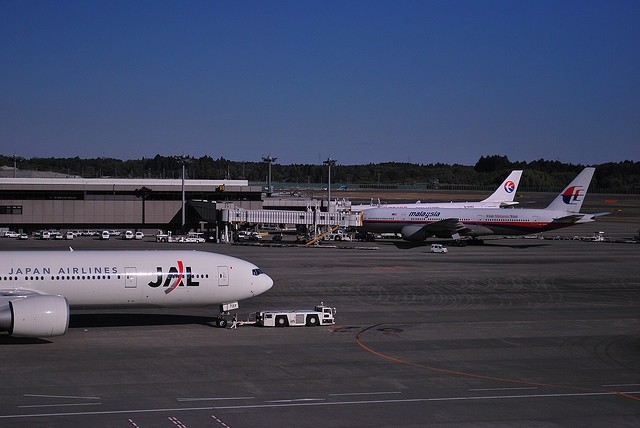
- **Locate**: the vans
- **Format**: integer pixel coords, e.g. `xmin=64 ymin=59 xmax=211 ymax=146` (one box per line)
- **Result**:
xmin=122 ymin=231 xmax=133 ymax=239
xmin=135 ymin=232 xmax=143 ymax=240
xmin=66 ymin=232 xmax=74 ymax=240
xmin=92 ymin=230 xmax=101 ymax=236
xmin=430 ymin=244 xmax=448 ymax=254
xmin=83 ymin=230 xmax=92 ymax=236
xmin=102 ymin=230 xmax=110 ymax=239
xmin=41 ymin=231 xmax=50 ymax=241
xmin=73 ymin=230 xmax=83 ymax=236
xmin=49 ymin=230 xmax=60 ymax=235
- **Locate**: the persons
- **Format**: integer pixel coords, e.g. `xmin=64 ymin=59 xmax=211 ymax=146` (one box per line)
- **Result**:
xmin=230 ymin=314 xmax=238 ymax=330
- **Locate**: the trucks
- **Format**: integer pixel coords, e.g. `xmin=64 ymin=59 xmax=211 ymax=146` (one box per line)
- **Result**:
xmin=374 ymin=232 xmax=402 ymax=239
xmin=157 ymin=230 xmax=205 ymax=244
xmin=323 ymin=230 xmax=353 ymax=241
xmin=255 ymin=301 xmax=338 ymax=328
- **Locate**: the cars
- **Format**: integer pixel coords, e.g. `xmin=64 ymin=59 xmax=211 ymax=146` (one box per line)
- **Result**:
xmin=55 ymin=234 xmax=63 ymax=239
xmin=33 ymin=232 xmax=42 ymax=241
xmin=479 ymin=231 xmax=606 ymax=241
xmin=238 ymin=230 xmax=262 ymax=242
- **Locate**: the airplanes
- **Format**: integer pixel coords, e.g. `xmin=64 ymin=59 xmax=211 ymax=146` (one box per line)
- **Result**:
xmin=362 ymin=168 xmax=611 ymax=243
xmin=351 ymin=170 xmax=526 ymax=212
xmin=0 ymin=248 xmax=274 ymax=338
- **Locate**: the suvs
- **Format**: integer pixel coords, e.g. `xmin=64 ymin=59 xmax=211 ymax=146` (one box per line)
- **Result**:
xmin=110 ymin=230 xmax=120 ymax=236
xmin=18 ymin=233 xmax=28 ymax=240
xmin=4 ymin=231 xmax=20 ymax=238
